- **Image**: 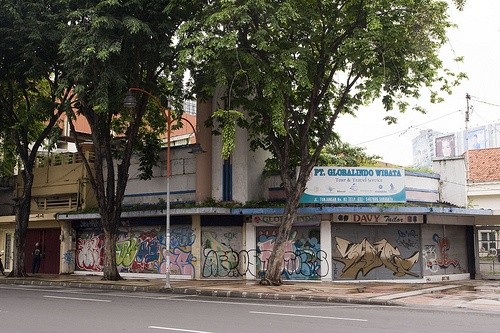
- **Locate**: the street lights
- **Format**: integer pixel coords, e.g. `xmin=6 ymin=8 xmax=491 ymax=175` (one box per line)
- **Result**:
xmin=122 ymin=88 xmax=207 ymax=290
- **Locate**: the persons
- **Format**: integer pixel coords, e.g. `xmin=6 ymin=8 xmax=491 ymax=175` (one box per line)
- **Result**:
xmin=32 ymin=241 xmax=42 ymax=274
